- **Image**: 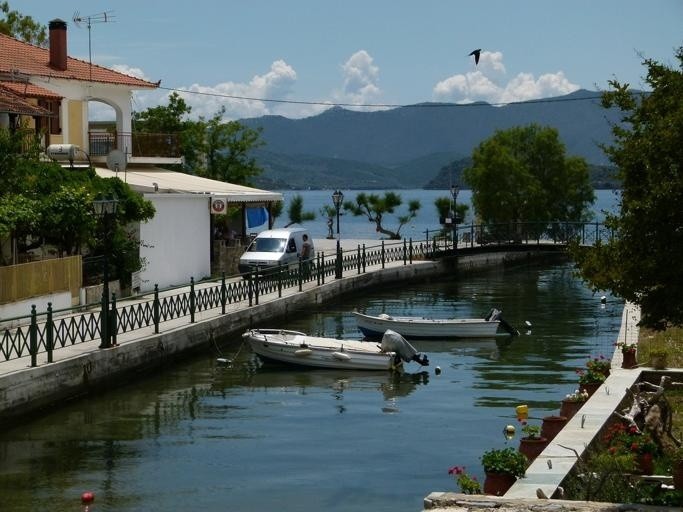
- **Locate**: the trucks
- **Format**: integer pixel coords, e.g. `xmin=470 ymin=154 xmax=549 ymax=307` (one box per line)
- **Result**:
xmin=234 ymin=226 xmax=317 ymax=282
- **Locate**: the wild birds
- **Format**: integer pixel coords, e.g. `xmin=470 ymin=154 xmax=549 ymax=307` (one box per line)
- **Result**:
xmin=468 ymin=49 xmax=482 ymax=65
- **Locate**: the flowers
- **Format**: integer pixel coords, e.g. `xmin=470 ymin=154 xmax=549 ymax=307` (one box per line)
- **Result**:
xmin=581 ymin=352 xmax=610 ymax=371
xmin=575 ymin=368 xmax=607 ymax=388
xmin=565 ymin=389 xmax=589 ymax=400
xmin=451 ymin=467 xmax=482 ymax=496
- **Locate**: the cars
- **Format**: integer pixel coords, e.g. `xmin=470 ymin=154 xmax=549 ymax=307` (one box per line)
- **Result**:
xmin=474 ymin=223 xmax=507 ymax=245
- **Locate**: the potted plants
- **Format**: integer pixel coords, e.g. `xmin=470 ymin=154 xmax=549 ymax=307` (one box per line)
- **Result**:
xmin=610 ymin=341 xmax=638 ymax=368
xmin=477 ymin=447 xmax=529 ymax=496
xmin=650 ymin=348 xmax=669 ymax=371
xmin=518 ymin=424 xmax=548 ymax=464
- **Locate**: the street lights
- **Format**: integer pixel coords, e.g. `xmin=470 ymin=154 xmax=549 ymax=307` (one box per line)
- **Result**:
xmin=330 ymin=187 xmax=345 ymax=277
xmin=87 ymin=189 xmax=120 ymax=348
xmin=448 ymin=180 xmax=461 ymax=259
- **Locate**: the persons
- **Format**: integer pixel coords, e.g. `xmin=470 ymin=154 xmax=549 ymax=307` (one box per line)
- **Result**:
xmin=300 ymin=233 xmax=312 ymax=280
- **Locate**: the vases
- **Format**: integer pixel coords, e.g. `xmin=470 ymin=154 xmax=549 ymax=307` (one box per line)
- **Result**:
xmin=580 ymin=382 xmax=602 ymax=395
xmin=559 ymin=400 xmax=580 ymax=423
xmin=603 ymin=371 xmax=611 ymax=377
xmin=541 ymin=415 xmax=567 ymax=441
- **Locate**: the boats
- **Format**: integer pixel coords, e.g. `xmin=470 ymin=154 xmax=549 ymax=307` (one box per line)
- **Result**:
xmin=362 ymin=336 xmax=507 ymax=362
xmin=241 ymin=323 xmax=417 ymax=376
xmin=217 ymin=365 xmax=433 ymax=401
xmin=349 ymin=307 xmax=520 ymax=341
